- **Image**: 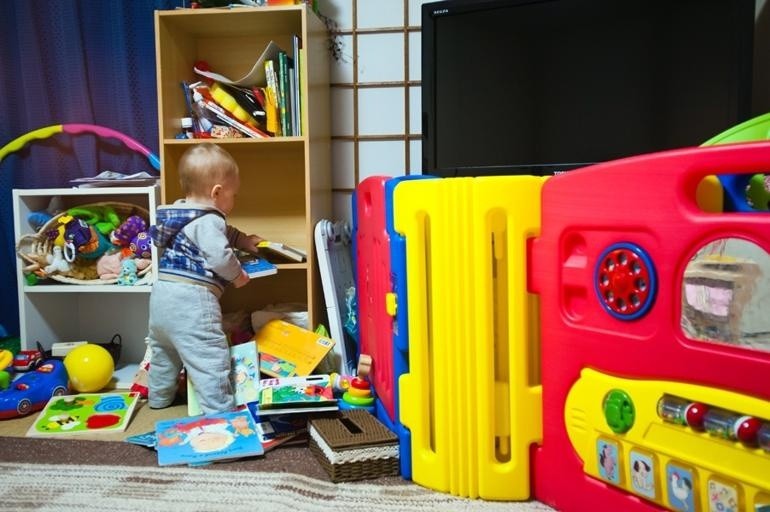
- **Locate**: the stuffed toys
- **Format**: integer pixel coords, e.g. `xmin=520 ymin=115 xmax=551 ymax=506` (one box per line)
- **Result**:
xmin=25 ymin=204 xmax=151 ymax=284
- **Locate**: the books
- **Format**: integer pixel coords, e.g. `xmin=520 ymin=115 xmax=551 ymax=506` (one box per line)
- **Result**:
xmin=227 ymin=241 xmax=307 ymax=281
xmin=125 ymin=401 xmax=303 ymax=467
xmin=185 ymin=319 xmax=338 ymax=417
xmin=104 ymin=362 xmax=142 ymax=390
xmin=25 ymin=392 xmax=140 ymax=432
xmin=183 ymin=33 xmax=304 ymax=137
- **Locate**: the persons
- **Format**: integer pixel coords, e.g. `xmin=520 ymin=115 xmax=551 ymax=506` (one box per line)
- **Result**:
xmin=148 ymin=143 xmax=263 ymax=415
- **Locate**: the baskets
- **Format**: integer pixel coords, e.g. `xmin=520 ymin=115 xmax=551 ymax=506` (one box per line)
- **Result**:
xmin=307 ymin=408 xmax=399 ymax=484
xmin=14 ymin=201 xmax=150 ymax=286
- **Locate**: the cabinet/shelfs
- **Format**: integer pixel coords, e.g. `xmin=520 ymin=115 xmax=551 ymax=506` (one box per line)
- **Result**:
xmin=13 ymin=180 xmax=160 ymax=390
xmin=151 ymin=5 xmax=332 ymax=344
